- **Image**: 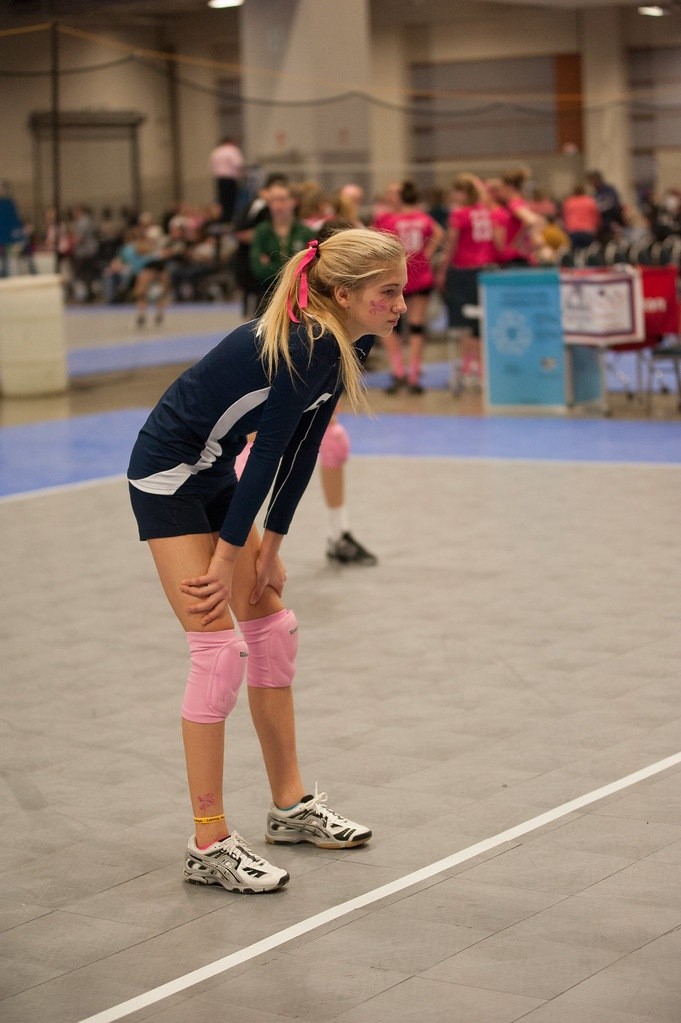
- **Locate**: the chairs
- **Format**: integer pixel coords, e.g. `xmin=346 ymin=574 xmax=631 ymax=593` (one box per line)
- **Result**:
xmin=0 ymin=212 xmax=680 ymax=329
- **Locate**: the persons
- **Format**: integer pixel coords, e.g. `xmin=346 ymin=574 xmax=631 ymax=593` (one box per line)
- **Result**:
xmin=374 ymin=179 xmax=447 ymax=396
xmin=209 ymin=134 xmax=265 ymax=219
xmin=126 ymin=229 xmax=408 ymax=895
xmin=227 ymin=213 xmax=384 ymax=570
xmin=439 ymin=166 xmax=512 ymax=405
xmin=0 ymin=172 xmax=679 ymax=334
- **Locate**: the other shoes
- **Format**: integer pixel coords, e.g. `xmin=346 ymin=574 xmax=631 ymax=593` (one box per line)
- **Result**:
xmin=449 ymin=375 xmax=481 ymax=397
xmin=386 ymin=377 xmax=424 ymax=395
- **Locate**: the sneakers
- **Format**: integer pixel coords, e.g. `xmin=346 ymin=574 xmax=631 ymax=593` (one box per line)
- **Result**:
xmin=327 ymin=531 xmax=377 ymax=566
xmin=266 ymin=780 xmax=372 ymax=850
xmin=183 ymin=832 xmax=290 ymax=894
